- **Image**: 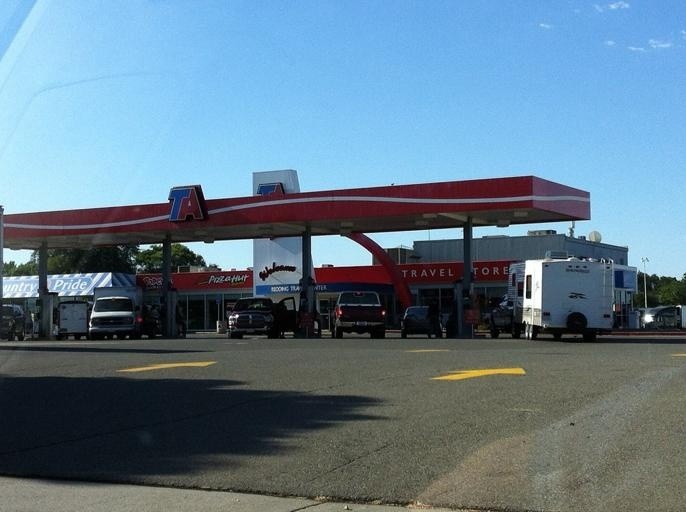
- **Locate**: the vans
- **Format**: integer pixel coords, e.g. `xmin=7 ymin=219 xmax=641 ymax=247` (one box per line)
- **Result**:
xmin=640 ymin=304 xmax=681 ymax=330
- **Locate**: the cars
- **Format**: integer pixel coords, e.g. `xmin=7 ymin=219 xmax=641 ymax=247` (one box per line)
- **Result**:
xmin=401 ymin=307 xmax=442 ymax=338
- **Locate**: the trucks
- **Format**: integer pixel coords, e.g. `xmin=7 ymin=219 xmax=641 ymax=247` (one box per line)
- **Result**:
xmin=58 ymin=301 xmax=89 ymax=340
xmin=88 ymin=287 xmax=142 ymax=339
xmin=507 ymin=255 xmax=615 ymax=342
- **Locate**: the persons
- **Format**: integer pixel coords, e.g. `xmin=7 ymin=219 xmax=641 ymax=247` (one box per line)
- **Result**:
xmin=25 ymin=308 xmax=59 ymax=335
xmin=136 ymin=304 xmax=161 ymax=319
xmin=427 ymin=299 xmax=442 ymax=338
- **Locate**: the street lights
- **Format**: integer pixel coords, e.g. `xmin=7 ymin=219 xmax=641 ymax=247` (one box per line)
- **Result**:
xmin=641 ymin=257 xmax=649 ymax=308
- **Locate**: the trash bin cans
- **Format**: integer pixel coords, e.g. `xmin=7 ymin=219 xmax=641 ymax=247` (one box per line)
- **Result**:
xmin=628 ymin=311 xmax=641 ymax=329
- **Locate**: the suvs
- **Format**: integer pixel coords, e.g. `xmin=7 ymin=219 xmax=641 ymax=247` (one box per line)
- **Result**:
xmin=227 ymin=297 xmax=296 ymax=338
xmin=332 ymin=292 xmax=386 ymax=339
xmin=0 ymin=304 xmax=27 ymax=341
